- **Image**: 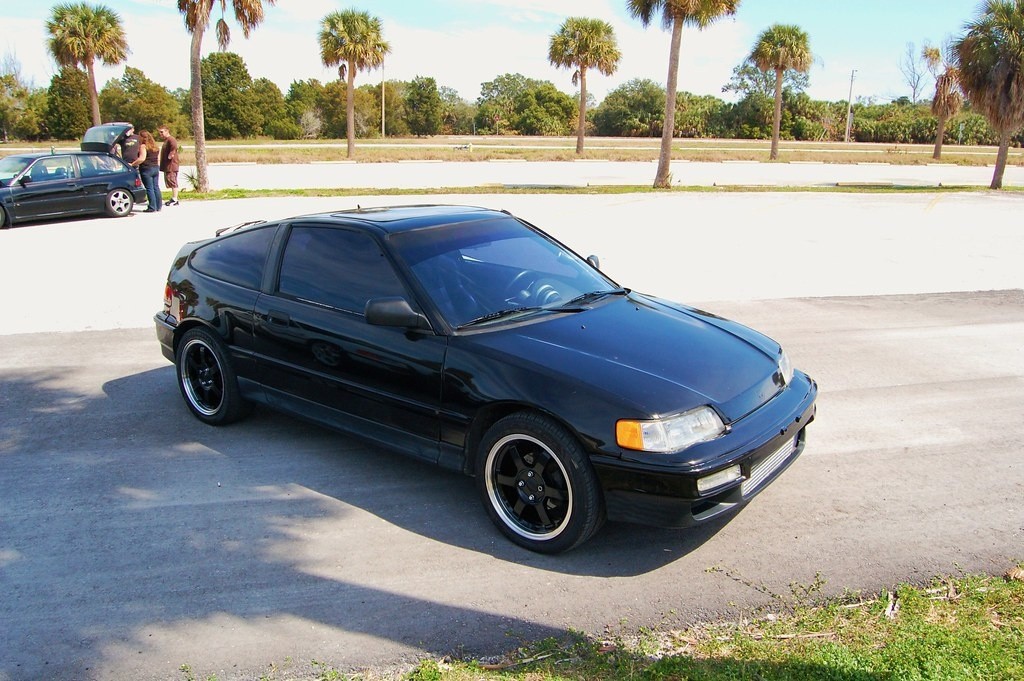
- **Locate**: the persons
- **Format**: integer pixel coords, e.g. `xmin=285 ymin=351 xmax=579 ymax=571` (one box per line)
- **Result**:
xmin=115 ymin=124 xmax=147 ymax=205
xmin=156 ymin=125 xmax=180 ymax=207
xmin=129 ymin=130 xmax=162 ymax=213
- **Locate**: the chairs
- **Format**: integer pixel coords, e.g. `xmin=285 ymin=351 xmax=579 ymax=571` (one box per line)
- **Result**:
xmin=298 ymin=250 xmax=479 ymax=320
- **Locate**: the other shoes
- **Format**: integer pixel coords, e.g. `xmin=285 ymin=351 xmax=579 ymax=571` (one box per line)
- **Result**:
xmin=143 ymin=208 xmax=155 ymax=212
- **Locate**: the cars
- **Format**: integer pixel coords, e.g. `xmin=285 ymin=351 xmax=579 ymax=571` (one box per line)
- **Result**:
xmin=154 ymin=204 xmax=822 ymax=558
xmin=0 ymin=121 xmax=145 ymax=230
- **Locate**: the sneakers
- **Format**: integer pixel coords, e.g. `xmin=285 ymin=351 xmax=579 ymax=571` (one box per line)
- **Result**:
xmin=165 ymin=198 xmax=179 ymax=207
